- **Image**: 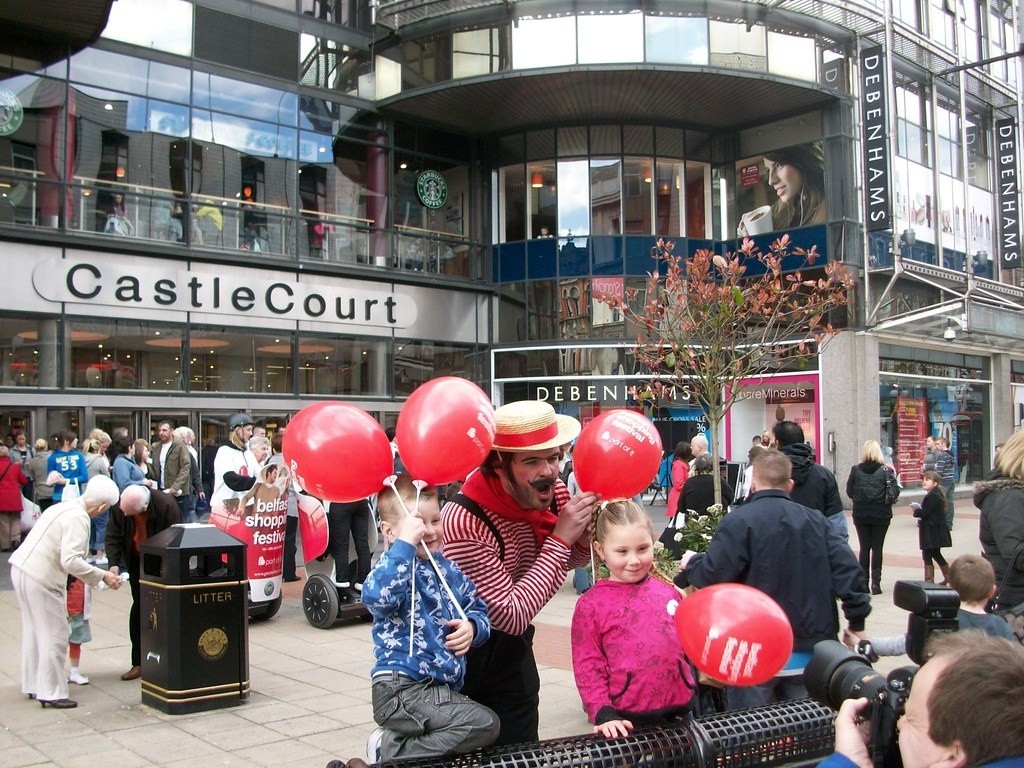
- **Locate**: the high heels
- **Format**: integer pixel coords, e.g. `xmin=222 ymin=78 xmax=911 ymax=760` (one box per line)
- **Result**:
xmin=872 ymin=583 xmax=883 ymax=594
xmin=38 ymin=694 xmax=78 ymax=710
xmin=28 ymin=690 xmax=38 ymax=699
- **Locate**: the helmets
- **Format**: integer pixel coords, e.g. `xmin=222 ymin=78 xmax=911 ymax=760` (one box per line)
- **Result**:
xmin=227 ymin=412 xmax=257 ymax=432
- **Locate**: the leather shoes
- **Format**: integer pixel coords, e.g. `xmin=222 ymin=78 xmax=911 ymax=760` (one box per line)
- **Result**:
xmin=121 ymin=664 xmax=143 ymax=681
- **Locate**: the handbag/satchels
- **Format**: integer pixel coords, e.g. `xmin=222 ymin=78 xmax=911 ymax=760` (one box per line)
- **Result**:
xmin=61 ymin=476 xmax=78 ymax=503
xmin=883 ymin=465 xmax=898 ymax=505
xmin=657 ymin=509 xmax=688 ymax=560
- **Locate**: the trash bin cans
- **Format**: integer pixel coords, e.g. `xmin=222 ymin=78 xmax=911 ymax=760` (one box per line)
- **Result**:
xmin=138 ymin=523 xmax=250 ymax=715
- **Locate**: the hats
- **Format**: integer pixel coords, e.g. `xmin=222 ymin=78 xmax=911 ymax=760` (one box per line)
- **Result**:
xmin=488 ymin=399 xmax=582 ymax=451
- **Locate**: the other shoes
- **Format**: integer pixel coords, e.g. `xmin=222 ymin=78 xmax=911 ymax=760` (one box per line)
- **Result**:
xmin=70 ymin=672 xmax=89 ymax=684
xmin=367 ymin=726 xmax=386 ymax=764
xmin=285 ymin=576 xmax=301 ymax=582
xmin=88 ymin=555 xmax=109 ymax=564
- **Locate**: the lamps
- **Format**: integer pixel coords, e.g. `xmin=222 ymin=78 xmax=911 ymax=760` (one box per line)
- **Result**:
xmin=898 ymin=228 xmax=915 ymax=249
xmin=971 ymin=250 xmax=988 ymax=268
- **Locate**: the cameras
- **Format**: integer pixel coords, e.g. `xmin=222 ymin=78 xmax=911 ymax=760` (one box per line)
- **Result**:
xmin=803 ymin=581 xmax=960 ymax=750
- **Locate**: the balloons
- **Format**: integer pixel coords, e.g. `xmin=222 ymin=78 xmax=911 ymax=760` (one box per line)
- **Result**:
xmin=573 ymin=408 xmax=663 ymax=500
xmin=394 ymin=377 xmax=495 ymax=487
xmin=284 ymin=399 xmax=397 ymax=504
xmin=674 ymin=584 xmax=794 ymax=687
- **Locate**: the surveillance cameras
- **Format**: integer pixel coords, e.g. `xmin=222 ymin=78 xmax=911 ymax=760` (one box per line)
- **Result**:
xmin=943 ymin=327 xmax=956 ymax=343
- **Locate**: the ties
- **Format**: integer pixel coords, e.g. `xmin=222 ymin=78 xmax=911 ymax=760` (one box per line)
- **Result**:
xmin=460 ymin=470 xmax=559 ymax=550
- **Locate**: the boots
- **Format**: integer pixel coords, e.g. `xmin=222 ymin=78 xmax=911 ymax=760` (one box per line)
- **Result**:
xmin=924 ymin=564 xmax=935 ymax=583
xmin=938 ymin=564 xmax=951 ymax=585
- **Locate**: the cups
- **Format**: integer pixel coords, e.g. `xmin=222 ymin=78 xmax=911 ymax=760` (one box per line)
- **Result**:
xmin=741 ymin=206 xmax=773 ymax=236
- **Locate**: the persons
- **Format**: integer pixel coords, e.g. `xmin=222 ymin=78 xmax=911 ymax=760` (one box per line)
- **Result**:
xmin=817 ymin=627 xmax=1023 ymax=768
xmin=557 ymin=441 xmax=595 ymax=595
xmin=8 ymin=430 xmax=37 ymax=501
xmin=919 ymin=433 xmax=956 ymax=531
xmin=666 ymin=434 xmax=735 ymax=527
xmin=7 ymin=475 xmax=123 ymax=707
xmin=47 ymin=430 xmax=88 ymax=504
xmin=236 ymin=462 xmax=282 ymax=517
xmin=671 ymin=451 xmax=871 ymax=754
xmin=26 ymin=439 xmax=55 ymax=510
xmin=147 ymin=418 xmax=207 ymax=522
xmin=384 ymin=428 xmax=410 ymax=472
xmin=248 ymin=425 xmax=373 ymax=603
xmin=910 ymin=470 xmax=954 ymax=586
xmin=103 ymin=482 xmax=181 ymax=681
xmin=79 ymin=426 xmax=157 ymax=564
xmin=736 ymin=139 xmax=826 ymax=237
xmin=435 ymin=396 xmax=602 ymax=768
xmin=845 ymin=440 xmax=899 ymax=595
xmin=209 ymin=409 xmax=267 ymax=516
xmin=366 ymin=475 xmax=500 ymax=760
xmin=316 ymin=213 xmax=334 ymax=259
xmin=971 ymin=426 xmax=1024 ymax=617
xmin=747 ymin=429 xmax=777 ymax=467
xmin=769 ymin=421 xmax=849 ymax=543
xmin=0 ymin=446 xmax=30 ymax=552
xmin=993 ymin=442 xmax=1007 ymax=455
xmin=537 ymin=226 xmax=553 ymax=239
xmin=842 ymin=551 xmax=1016 ymax=660
xmin=107 ymin=186 xmax=221 ymax=246
xmin=574 ymin=493 xmax=703 ymax=768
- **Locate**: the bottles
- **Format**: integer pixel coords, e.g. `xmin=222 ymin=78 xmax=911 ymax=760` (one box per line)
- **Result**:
xmin=885 ymin=481 xmax=891 ymax=494
